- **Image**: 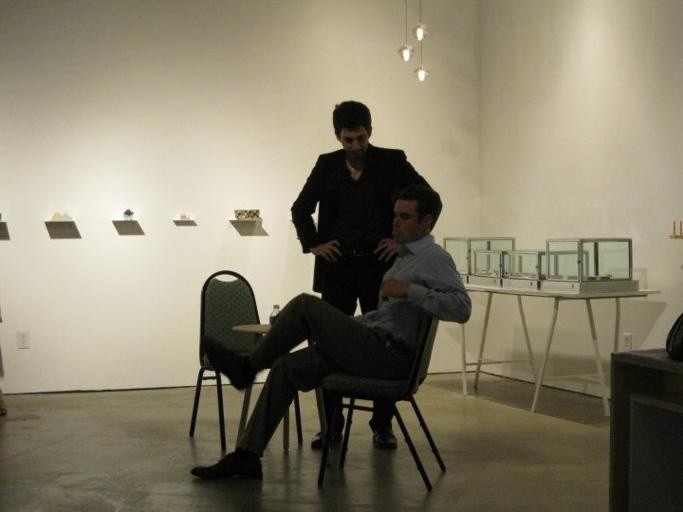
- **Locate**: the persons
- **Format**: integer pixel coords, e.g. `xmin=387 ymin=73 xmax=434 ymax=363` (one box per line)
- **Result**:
xmin=182 ymin=180 xmax=472 ymax=479
xmin=288 ymin=98 xmax=445 ymax=449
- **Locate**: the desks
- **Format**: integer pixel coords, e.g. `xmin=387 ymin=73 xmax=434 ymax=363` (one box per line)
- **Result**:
xmin=232 ymin=322 xmax=333 ymax=457
xmin=443 ymin=285 xmax=662 ymax=419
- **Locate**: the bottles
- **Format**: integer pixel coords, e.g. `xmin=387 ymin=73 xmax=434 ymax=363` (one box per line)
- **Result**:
xmin=124 ymin=209 xmax=131 ymax=221
xmin=269 ymin=304 xmax=279 ymax=329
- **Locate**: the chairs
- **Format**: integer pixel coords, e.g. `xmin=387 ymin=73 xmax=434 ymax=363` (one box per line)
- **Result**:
xmin=312 ymin=308 xmax=450 ymax=496
xmin=184 ymin=269 xmax=306 ymax=456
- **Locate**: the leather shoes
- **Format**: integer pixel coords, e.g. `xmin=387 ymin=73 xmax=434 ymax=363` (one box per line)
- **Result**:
xmin=311 ymin=430 xmax=342 ymax=449
xmin=190 ymin=450 xmax=263 ymax=480
xmin=205 ymin=338 xmax=256 ymax=390
xmin=372 ymin=429 xmax=395 ymax=449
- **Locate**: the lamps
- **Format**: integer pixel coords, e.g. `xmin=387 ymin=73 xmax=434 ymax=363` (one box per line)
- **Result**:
xmin=396 ymin=0 xmax=433 ymax=83
